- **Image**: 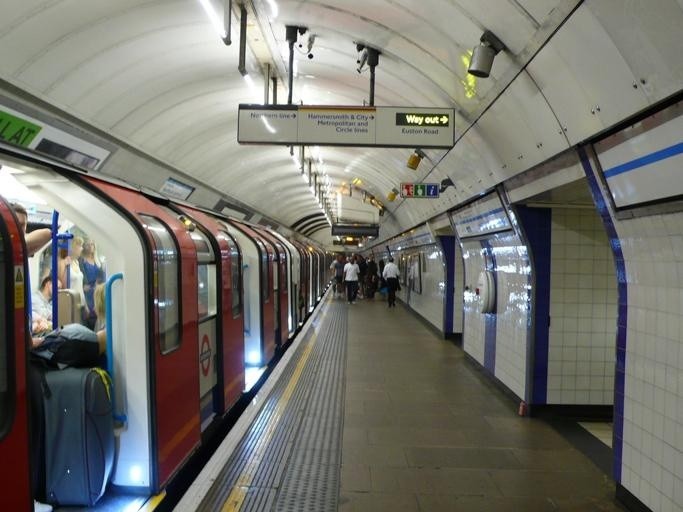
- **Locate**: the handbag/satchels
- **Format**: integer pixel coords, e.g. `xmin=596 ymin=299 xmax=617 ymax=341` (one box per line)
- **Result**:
xmin=36 ymin=322 xmax=100 ymax=367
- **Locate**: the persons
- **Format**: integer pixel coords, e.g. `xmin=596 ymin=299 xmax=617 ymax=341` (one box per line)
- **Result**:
xmin=29 ymin=234 xmax=111 ymax=370
xmin=10 ymin=202 xmax=75 ymax=256
xmin=329 ymin=254 xmax=399 ymax=307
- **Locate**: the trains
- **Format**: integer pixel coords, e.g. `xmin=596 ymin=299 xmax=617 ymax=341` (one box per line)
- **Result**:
xmin=0 ymin=143 xmax=339 ymax=511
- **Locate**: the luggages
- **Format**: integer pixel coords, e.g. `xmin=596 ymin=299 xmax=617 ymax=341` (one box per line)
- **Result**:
xmin=40 ymin=365 xmax=116 ymax=509
